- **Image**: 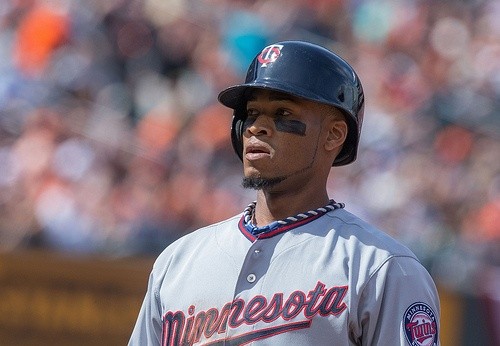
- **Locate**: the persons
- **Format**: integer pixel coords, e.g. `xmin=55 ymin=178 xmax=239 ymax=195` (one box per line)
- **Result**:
xmin=125 ymin=43 xmax=442 ymax=345
xmin=1 ymin=0 xmax=500 ymax=302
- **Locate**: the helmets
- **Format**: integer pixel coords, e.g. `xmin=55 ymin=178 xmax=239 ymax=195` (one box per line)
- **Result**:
xmin=218 ymin=41 xmax=365 ymax=167
